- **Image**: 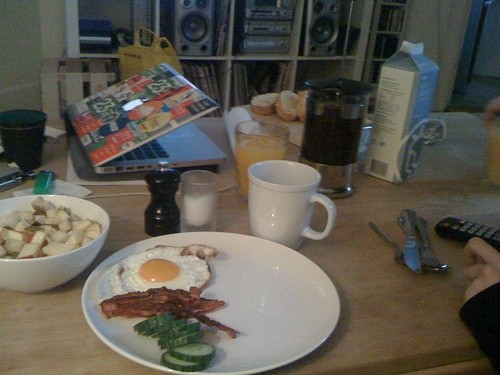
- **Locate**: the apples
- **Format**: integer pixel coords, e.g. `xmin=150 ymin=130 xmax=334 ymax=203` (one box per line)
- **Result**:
xmin=0 ymin=197 xmax=102 ymax=260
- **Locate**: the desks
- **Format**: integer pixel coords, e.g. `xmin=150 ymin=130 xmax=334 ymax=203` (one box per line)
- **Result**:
xmin=0 ymin=110 xmax=500 ymax=375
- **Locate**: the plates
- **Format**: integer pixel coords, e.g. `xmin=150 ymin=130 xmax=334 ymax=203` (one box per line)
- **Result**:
xmin=79 ymin=230 xmax=342 ymax=375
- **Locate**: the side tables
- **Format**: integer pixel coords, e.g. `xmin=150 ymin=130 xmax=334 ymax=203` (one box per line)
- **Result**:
xmin=64 ymin=0 xmax=375 ymax=119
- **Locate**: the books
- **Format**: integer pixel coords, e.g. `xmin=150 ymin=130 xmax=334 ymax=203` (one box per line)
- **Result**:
xmin=184 ymin=62 xmax=223 ymax=118
xmin=232 ymin=62 xmax=249 ymax=106
xmin=270 ymin=61 xmax=291 ymax=92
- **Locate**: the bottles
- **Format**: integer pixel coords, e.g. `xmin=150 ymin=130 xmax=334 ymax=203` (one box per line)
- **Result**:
xmin=143 ymin=168 xmax=180 ymax=236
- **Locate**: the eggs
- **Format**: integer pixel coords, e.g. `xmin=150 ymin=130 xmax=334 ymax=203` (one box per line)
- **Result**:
xmin=109 ymin=243 xmax=219 ymax=292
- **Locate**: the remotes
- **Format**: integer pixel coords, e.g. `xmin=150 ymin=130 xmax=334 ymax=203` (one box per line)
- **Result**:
xmin=435 ymin=215 xmax=500 ymax=253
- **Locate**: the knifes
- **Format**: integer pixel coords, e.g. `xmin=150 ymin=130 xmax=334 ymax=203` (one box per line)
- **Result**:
xmin=396 ymin=209 xmax=424 ymax=274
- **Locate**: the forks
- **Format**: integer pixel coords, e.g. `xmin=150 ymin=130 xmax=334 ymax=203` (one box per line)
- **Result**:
xmin=415 ymin=216 xmax=450 ymax=272
xmin=368 ymin=220 xmax=407 ymax=265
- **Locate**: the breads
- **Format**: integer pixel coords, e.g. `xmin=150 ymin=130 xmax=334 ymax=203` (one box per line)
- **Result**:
xmin=250 ymin=90 xmax=308 ymax=121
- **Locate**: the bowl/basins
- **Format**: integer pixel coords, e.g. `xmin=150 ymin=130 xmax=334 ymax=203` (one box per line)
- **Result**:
xmin=0 ymin=194 xmax=111 ymax=292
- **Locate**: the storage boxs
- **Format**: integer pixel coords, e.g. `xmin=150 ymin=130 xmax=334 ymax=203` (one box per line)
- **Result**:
xmin=79 ymin=9 xmax=113 ymax=30
xmin=39 ymin=56 xmax=116 ymax=121
xmin=79 ymin=31 xmax=112 ymax=54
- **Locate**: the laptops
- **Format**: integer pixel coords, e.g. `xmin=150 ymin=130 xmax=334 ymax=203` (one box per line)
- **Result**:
xmin=64 ymin=62 xmax=226 ymax=175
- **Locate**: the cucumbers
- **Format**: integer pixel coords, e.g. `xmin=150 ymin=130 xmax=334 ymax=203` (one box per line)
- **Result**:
xmin=132 ymin=311 xmax=204 ymax=349
xmin=160 ymin=342 xmax=216 ymax=372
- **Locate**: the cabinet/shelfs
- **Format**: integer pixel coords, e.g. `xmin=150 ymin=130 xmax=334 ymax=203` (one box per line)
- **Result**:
xmin=352 ymin=0 xmax=408 ymax=115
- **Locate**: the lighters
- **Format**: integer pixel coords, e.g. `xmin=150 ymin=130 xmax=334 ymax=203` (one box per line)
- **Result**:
xmin=0 ymin=172 xmax=24 ymax=192
xmin=34 ymin=170 xmax=54 ymax=194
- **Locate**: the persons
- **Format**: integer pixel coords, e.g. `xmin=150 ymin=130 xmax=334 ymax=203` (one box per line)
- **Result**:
xmin=458 ymin=237 xmax=500 ymax=375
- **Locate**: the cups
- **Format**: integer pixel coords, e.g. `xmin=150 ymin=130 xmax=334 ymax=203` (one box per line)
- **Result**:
xmin=296 ymin=76 xmax=383 ymax=200
xmin=247 ymin=160 xmax=337 ymax=250
xmin=179 ymin=170 xmax=223 ymax=232
xmin=233 ymin=120 xmax=291 ymax=198
xmin=0 ymin=109 xmax=49 ymax=170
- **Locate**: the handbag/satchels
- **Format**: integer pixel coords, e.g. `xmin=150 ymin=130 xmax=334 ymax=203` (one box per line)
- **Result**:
xmin=118 ymin=27 xmax=184 ymax=81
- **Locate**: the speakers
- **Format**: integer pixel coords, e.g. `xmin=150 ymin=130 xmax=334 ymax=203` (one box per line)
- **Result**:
xmin=303 ymin=0 xmax=342 ymax=57
xmin=166 ymin=0 xmax=215 ymax=56
